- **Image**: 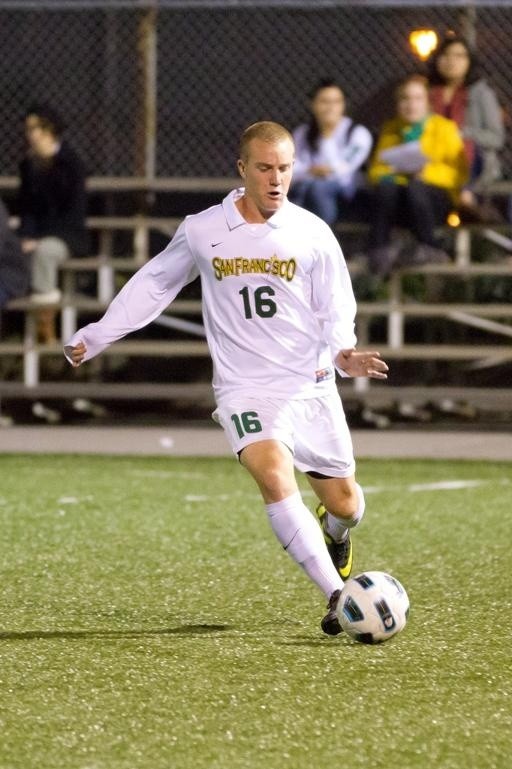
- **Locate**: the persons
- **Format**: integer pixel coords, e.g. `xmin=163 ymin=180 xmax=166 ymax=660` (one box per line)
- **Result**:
xmin=368 ymin=63 xmax=466 ymax=273
xmin=3 ymin=104 xmax=93 ymax=343
xmin=287 ymin=76 xmax=374 ymax=228
xmin=426 ymin=35 xmax=509 ymax=198
xmin=68 ymin=118 xmax=387 ymax=636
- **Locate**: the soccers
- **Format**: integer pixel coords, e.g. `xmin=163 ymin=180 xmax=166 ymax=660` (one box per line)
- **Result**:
xmin=335 ymin=572 xmax=410 ymax=644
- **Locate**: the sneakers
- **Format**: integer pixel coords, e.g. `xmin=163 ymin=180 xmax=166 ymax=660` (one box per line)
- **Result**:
xmin=314 ymin=500 xmax=354 ymax=637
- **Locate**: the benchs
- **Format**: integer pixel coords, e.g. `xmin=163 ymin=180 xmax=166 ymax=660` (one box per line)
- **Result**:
xmin=0 ymin=173 xmax=512 ymax=413
